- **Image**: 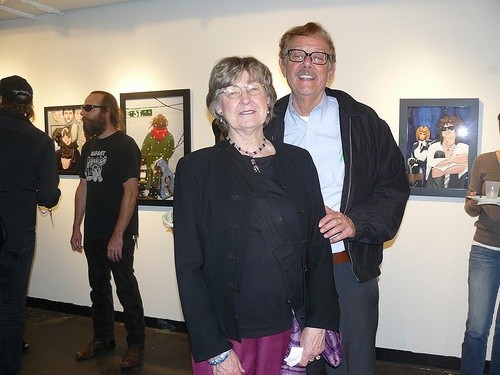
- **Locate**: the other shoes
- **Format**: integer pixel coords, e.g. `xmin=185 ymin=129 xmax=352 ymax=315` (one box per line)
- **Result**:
xmin=76 ymin=340 xmax=116 ymax=360
xmin=121 ymin=345 xmax=144 ymax=370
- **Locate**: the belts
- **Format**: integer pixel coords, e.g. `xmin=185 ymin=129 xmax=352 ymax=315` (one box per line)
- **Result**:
xmin=333 ymin=252 xmax=351 ymax=265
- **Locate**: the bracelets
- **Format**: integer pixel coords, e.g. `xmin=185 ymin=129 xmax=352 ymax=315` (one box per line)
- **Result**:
xmin=207 ymin=349 xmax=232 ymax=366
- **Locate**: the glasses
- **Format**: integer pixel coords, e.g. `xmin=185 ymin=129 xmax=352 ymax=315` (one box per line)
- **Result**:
xmin=285 ymin=49 xmax=332 ymax=65
xmin=81 ymin=105 xmax=113 ymax=112
xmin=218 ymin=83 xmax=267 ymax=97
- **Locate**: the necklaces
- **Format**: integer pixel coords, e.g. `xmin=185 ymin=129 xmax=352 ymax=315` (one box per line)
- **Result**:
xmin=226 ymin=135 xmax=267 ymax=171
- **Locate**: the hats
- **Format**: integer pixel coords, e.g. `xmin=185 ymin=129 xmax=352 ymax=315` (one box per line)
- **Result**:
xmin=0 ymin=75 xmax=33 ymax=105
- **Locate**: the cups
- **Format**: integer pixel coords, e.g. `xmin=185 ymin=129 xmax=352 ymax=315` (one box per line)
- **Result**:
xmin=485 ymin=181 xmax=500 ymax=198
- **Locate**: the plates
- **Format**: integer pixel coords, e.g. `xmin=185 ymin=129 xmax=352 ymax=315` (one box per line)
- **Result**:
xmin=467 ymin=195 xmax=500 ymax=202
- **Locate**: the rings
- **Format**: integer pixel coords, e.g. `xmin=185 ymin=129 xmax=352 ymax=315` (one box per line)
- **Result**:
xmin=316 ymin=356 xmax=320 ymax=360
xmin=309 ymin=358 xmax=314 ymax=362
xmin=115 ymin=253 xmax=118 ymax=256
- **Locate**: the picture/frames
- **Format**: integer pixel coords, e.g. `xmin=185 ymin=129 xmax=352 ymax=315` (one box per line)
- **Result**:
xmin=44 ymin=105 xmax=83 ymax=175
xmin=399 ymin=98 xmax=479 ymax=197
xmin=120 ymin=89 xmax=192 ymax=207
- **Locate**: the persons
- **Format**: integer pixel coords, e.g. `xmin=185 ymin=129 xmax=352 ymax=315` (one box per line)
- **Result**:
xmin=459 ymin=113 xmax=500 ymax=375
xmin=0 ymin=75 xmax=145 ymax=375
xmin=212 ymin=22 xmax=409 ymax=375
xmin=174 ymin=56 xmax=340 ymax=375
